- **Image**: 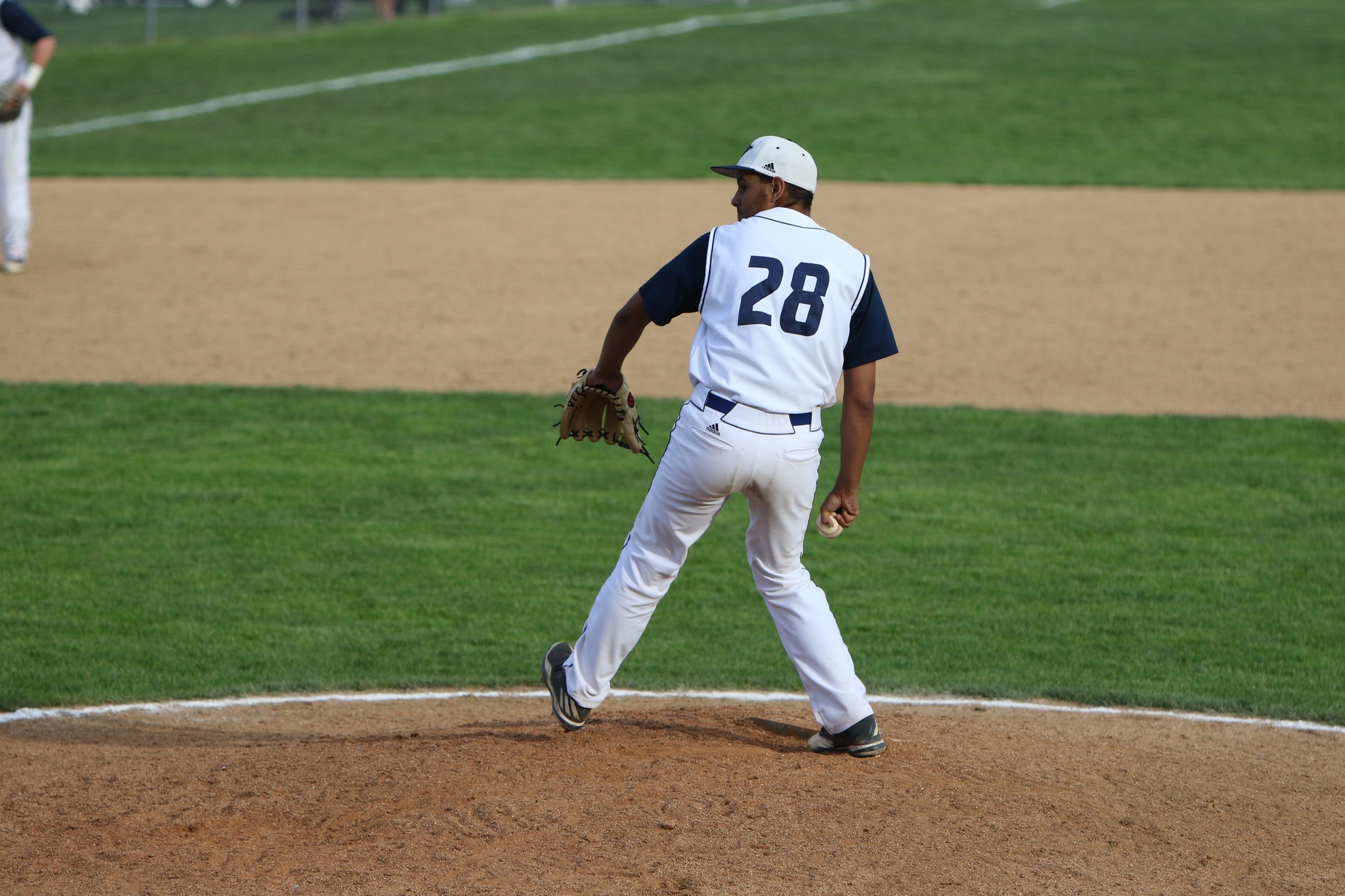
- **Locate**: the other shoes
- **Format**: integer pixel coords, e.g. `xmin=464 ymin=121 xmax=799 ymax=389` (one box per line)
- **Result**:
xmin=2 ymin=259 xmax=25 ymax=275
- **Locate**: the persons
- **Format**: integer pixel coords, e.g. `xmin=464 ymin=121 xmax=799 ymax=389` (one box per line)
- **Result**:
xmin=0 ymin=0 xmax=56 ymax=273
xmin=69 ymin=0 xmax=453 ymax=21
xmin=540 ymin=133 xmax=900 ymax=756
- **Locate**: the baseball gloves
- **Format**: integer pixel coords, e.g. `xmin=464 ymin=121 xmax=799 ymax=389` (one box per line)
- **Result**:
xmin=0 ymin=79 xmax=31 ymax=124
xmin=559 ymin=366 xmax=642 ymax=454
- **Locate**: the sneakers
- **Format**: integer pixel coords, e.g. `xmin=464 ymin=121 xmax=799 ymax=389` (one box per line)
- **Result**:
xmin=541 ymin=642 xmax=592 ymax=733
xmin=807 ymin=715 xmax=886 ymax=758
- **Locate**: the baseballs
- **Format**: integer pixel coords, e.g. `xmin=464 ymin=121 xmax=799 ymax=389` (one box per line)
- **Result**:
xmin=815 ymin=510 xmax=843 ymax=540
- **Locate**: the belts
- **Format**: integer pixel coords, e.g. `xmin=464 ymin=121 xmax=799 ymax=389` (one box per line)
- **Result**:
xmin=704 ymin=391 xmax=811 ymax=427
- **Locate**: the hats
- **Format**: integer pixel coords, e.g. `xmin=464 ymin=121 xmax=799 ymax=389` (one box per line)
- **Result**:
xmin=710 ymin=136 xmax=817 ymax=196
